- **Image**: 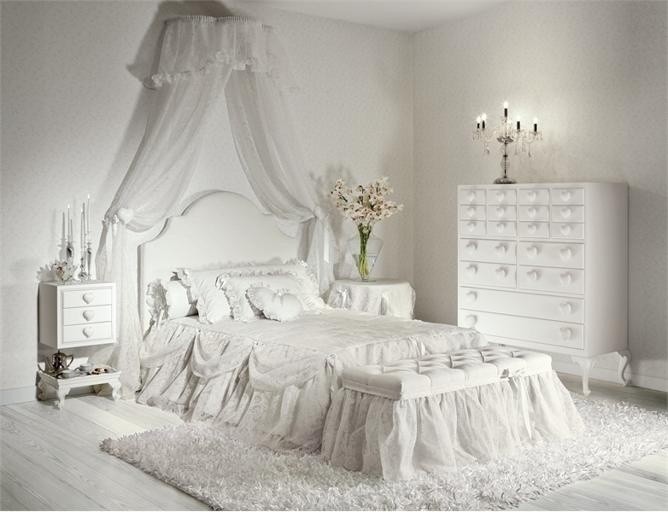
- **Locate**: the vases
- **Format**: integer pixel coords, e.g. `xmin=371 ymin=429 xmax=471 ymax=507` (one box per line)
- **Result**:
xmin=347 ymin=229 xmax=384 ymax=283
xmin=55 ymin=270 xmax=74 ymax=284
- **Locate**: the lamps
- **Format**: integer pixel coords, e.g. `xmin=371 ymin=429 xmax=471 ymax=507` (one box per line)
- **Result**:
xmin=473 ymin=99 xmax=542 ymax=184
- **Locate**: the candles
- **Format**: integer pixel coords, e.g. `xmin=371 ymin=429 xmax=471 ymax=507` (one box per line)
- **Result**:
xmin=61 ymin=194 xmax=91 ymax=250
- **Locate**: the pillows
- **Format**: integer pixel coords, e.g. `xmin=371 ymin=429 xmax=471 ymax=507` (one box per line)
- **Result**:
xmin=147 ymin=255 xmax=330 ymax=324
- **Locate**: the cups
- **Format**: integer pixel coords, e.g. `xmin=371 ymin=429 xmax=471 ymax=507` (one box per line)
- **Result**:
xmin=56 ymin=370 xmax=74 ymax=380
xmin=75 ymin=363 xmax=90 ymax=373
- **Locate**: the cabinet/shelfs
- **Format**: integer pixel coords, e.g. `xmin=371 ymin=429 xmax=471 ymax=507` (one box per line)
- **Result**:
xmin=456 ymin=184 xmax=633 ymax=397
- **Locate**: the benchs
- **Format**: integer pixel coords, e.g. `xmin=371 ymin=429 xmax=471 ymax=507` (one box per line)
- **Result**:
xmin=337 ymin=344 xmax=552 ymax=476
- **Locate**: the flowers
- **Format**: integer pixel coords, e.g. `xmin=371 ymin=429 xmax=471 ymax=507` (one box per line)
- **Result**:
xmin=331 ymin=175 xmax=404 ymax=279
xmin=52 ymin=255 xmax=79 ymax=273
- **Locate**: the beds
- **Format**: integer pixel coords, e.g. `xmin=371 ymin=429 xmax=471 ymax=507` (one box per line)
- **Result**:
xmin=139 ymin=190 xmax=485 ymax=450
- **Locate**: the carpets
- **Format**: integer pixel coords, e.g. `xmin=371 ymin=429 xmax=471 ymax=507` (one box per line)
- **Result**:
xmin=99 ymin=386 xmax=667 ymax=511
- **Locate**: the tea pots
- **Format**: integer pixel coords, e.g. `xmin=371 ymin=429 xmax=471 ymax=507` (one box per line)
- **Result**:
xmin=46 ymin=349 xmax=74 ymax=371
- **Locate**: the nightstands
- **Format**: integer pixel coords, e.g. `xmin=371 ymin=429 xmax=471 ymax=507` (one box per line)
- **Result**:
xmin=39 ymin=280 xmax=117 ymax=351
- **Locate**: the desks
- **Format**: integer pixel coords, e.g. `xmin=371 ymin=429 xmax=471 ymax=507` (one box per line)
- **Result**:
xmin=36 ymin=364 xmax=123 ymax=410
xmin=332 ymin=280 xmax=411 ymax=318
